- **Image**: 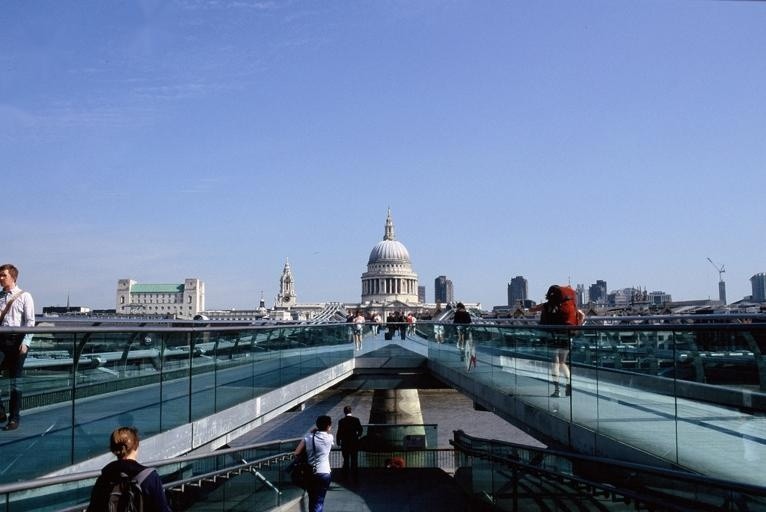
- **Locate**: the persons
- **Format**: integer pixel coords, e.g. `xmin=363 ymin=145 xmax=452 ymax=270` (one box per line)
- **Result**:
xmin=336 ymin=405 xmax=363 ymax=479
xmin=85 ymin=426 xmax=174 ymax=511
xmin=520 ymin=285 xmax=586 ymax=398
xmin=293 ymin=414 xmax=335 ymax=511
xmin=453 ymin=303 xmax=472 ymax=350
xmin=346 ymin=308 xmax=418 ymax=350
xmin=0 ymin=264 xmax=35 ymax=430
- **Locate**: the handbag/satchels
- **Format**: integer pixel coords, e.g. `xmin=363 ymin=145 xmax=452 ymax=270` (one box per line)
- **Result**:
xmin=291 ymin=463 xmax=313 ymax=487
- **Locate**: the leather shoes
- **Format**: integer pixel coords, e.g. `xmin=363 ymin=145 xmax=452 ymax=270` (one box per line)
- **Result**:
xmin=0 ymin=413 xmax=7 ymax=423
xmin=6 ymin=420 xmax=18 ymax=429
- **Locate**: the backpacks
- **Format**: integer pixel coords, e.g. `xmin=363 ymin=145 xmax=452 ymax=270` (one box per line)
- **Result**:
xmin=548 ymin=285 xmax=580 ymax=334
xmin=106 ymin=466 xmax=155 ymax=512
